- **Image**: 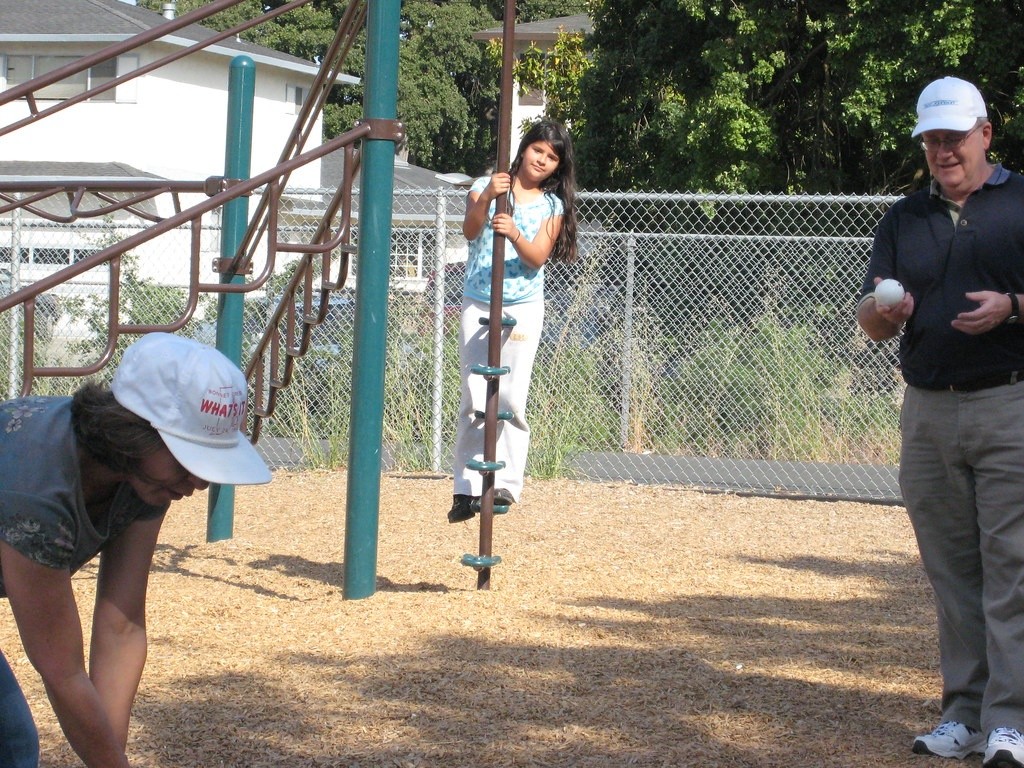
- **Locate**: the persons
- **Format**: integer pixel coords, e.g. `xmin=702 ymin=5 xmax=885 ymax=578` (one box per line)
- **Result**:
xmin=447 ymin=119 xmax=578 ymax=523
xmin=856 ymin=77 xmax=1024 ymax=768
xmin=0 ymin=331 xmax=272 ymax=768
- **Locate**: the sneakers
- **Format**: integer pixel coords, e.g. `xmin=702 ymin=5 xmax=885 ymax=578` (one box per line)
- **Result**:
xmin=447 ymin=494 xmax=476 ymax=523
xmin=910 ymin=719 xmax=987 ymax=761
xmin=493 ymin=488 xmax=516 ymax=506
xmin=982 ymin=726 xmax=1024 ymax=768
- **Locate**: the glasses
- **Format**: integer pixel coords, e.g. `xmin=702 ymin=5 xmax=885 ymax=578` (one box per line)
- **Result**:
xmin=918 ymin=123 xmax=984 ymax=152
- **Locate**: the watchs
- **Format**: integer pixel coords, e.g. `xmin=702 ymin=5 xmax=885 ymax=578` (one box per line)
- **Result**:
xmin=1005 ymin=292 xmax=1019 ymax=326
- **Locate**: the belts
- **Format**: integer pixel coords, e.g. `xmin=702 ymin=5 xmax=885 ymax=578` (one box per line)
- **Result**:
xmin=943 ymin=369 xmax=1024 ymax=392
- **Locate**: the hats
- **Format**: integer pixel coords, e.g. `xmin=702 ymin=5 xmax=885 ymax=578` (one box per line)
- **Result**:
xmin=910 ymin=75 xmax=989 ymax=139
xmin=110 ymin=331 xmax=274 ymax=486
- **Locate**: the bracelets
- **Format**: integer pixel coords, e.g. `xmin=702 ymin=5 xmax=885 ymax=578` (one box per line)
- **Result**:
xmin=510 ymin=230 xmax=520 ymax=243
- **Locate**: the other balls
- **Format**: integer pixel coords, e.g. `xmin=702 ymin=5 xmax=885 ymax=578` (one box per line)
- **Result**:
xmin=874 ymin=278 xmax=904 ymax=308
